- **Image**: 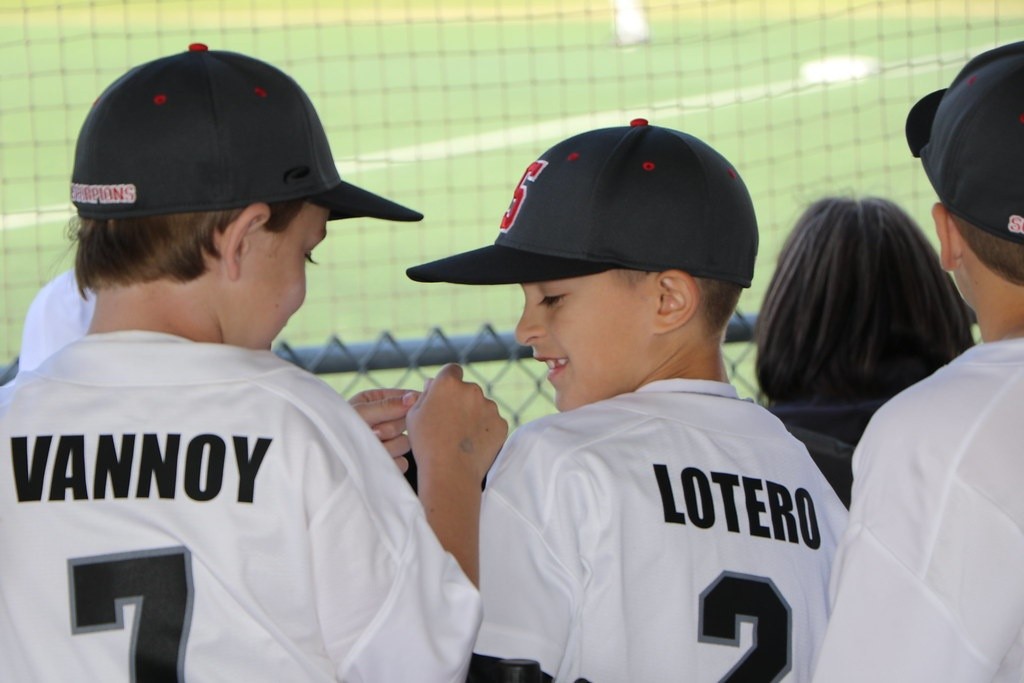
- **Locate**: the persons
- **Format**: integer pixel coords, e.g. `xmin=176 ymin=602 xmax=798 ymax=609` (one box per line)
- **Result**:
xmin=814 ymin=40 xmax=1024 ymax=683
xmin=464 ymin=119 xmax=852 ymax=683
xmin=753 ymin=194 xmax=977 ymax=512
xmin=1 ymin=44 xmax=511 ymax=682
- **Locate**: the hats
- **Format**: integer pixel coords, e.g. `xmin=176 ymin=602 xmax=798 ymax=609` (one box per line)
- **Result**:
xmin=904 ymin=40 xmax=1024 ymax=244
xmin=407 ymin=118 xmax=760 ymax=288
xmin=69 ymin=43 xmax=424 ymax=221
